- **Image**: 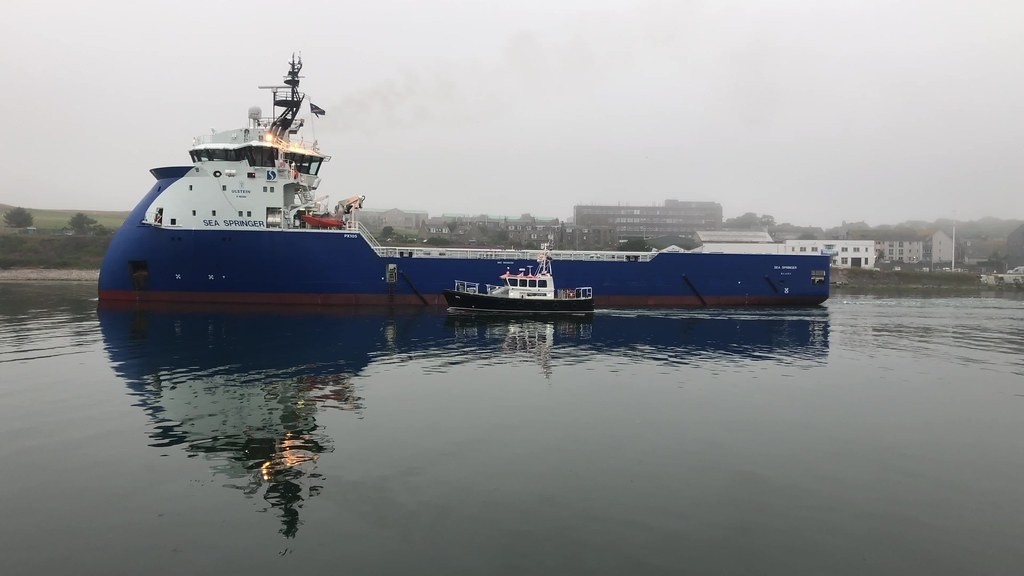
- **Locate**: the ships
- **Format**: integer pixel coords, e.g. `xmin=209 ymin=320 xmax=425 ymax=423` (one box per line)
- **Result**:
xmin=96 ymin=44 xmax=833 ymax=314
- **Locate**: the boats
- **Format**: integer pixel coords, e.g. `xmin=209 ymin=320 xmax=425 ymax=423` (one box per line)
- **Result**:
xmin=439 ymin=232 xmax=595 ymax=317
xmin=976 ymin=265 xmax=1024 ymax=287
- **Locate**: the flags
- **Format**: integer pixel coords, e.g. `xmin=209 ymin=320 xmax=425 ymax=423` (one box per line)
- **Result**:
xmin=309 ymin=103 xmax=325 ymax=117
